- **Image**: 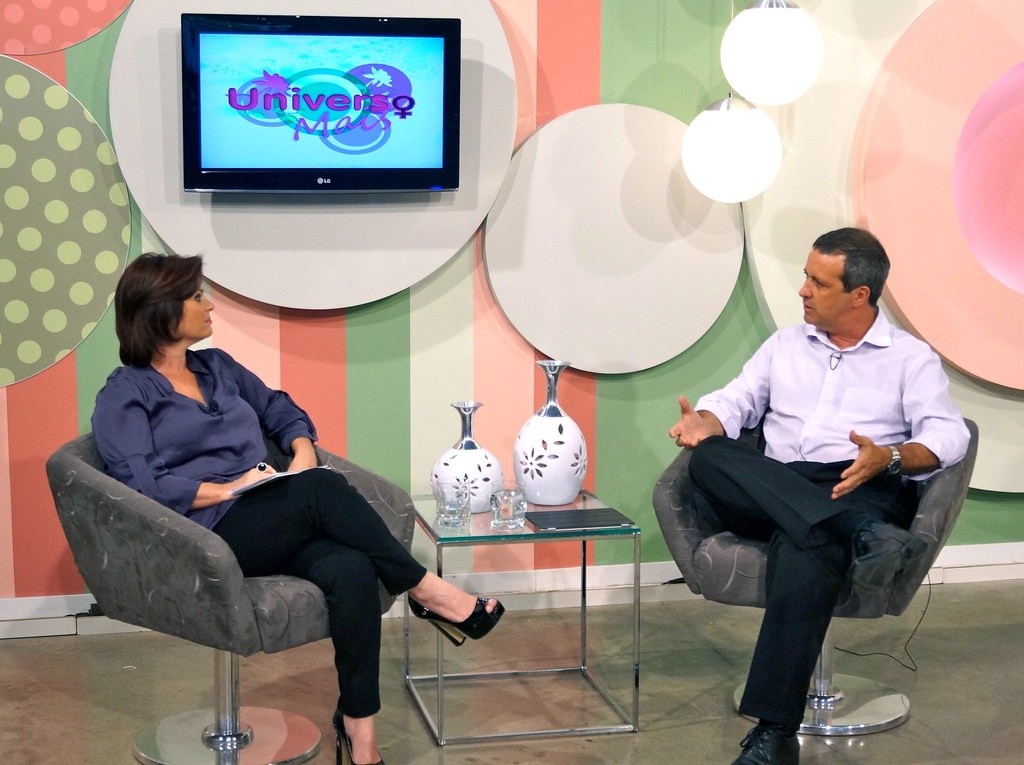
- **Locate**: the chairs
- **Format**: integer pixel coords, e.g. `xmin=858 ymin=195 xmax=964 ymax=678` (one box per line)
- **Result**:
xmin=47 ymin=433 xmax=413 ymax=765
xmin=652 ymin=415 xmax=980 ymax=737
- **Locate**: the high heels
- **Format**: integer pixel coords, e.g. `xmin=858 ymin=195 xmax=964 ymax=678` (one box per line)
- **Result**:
xmin=332 ymin=706 xmax=386 ymax=765
xmin=408 ymin=581 xmax=505 ymax=646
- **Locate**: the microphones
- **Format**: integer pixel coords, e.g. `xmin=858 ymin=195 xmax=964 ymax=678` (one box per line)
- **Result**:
xmin=209 ymin=399 xmax=223 ymax=415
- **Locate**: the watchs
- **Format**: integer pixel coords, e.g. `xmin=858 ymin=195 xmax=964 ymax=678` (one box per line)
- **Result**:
xmin=887 ymin=446 xmax=903 ymax=475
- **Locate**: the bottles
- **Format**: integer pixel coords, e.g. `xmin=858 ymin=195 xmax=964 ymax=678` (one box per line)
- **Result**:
xmin=431 ymin=401 xmax=504 ymax=515
xmin=513 ymin=359 xmax=588 ymax=506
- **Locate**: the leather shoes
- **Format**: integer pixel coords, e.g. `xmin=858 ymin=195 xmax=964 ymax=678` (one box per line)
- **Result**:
xmin=847 ymin=523 xmax=926 ymax=594
xmin=731 ymin=727 xmax=801 ymax=765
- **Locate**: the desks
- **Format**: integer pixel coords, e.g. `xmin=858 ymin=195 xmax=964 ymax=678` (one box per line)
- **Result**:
xmin=402 ymin=492 xmax=642 ymax=745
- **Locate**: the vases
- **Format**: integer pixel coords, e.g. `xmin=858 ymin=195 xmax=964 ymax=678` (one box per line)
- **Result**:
xmin=431 ymin=400 xmax=504 ymax=512
xmin=513 ymin=360 xmax=588 ymax=504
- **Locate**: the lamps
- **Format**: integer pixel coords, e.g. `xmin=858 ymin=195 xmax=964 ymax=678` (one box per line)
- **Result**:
xmin=680 ymin=0 xmax=824 ymax=204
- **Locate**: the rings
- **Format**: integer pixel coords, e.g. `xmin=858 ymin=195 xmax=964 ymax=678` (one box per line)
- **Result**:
xmin=256 ymin=462 xmax=267 ymax=473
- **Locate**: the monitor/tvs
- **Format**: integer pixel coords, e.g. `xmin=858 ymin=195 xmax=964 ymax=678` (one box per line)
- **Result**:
xmin=181 ymin=13 xmax=462 ymax=193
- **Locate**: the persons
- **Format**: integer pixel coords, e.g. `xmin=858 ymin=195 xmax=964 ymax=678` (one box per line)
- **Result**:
xmin=668 ymin=228 xmax=971 ymax=765
xmin=91 ymin=252 xmax=505 ymax=765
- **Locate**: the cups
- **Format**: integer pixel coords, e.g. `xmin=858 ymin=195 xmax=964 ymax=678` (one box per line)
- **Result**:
xmin=488 ymin=480 xmax=527 ymax=530
xmin=429 ymin=480 xmax=471 ymax=527
